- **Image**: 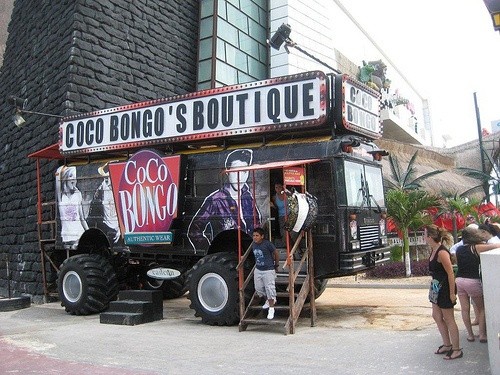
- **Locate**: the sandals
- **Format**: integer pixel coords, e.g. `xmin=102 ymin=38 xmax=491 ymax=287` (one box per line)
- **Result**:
xmin=434 ymin=343 xmax=464 ymax=360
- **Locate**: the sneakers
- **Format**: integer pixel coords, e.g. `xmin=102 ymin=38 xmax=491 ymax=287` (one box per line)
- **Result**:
xmin=262 ymin=299 xmax=275 ymax=320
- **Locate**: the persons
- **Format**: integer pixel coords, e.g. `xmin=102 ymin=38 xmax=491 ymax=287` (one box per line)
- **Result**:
xmin=55 ymin=166 xmax=90 ymax=245
xmin=251 ymin=227 xmax=280 ymax=320
xmin=187 ymin=148 xmax=262 ymax=254
xmin=86 ymin=160 xmax=125 ymax=246
xmin=450 ymin=223 xmax=500 ymax=325
xmin=424 ymin=225 xmax=464 ymax=360
xmin=271 ymin=181 xmax=297 ymax=248
xmin=456 ymin=227 xmax=500 ymax=343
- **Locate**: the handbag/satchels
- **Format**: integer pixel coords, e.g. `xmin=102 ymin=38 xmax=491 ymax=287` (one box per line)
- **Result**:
xmin=437 ymin=288 xmax=456 ymax=309
xmin=472 ymin=245 xmax=482 ymax=284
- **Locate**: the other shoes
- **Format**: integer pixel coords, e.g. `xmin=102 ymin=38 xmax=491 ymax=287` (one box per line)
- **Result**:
xmin=479 ymin=337 xmax=487 ymax=343
xmin=471 ymin=319 xmax=479 ymax=326
xmin=466 ymin=337 xmax=475 ymax=342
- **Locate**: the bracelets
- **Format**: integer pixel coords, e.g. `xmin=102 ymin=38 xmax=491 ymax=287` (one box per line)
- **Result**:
xmin=274 ymin=264 xmax=279 ymax=267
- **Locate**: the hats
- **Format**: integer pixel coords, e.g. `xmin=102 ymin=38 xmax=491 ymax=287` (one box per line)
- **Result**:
xmin=97 ymin=161 xmax=120 ymax=177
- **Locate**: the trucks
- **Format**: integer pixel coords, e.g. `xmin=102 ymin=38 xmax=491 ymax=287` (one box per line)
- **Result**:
xmin=55 ymin=69 xmax=391 ymax=326
